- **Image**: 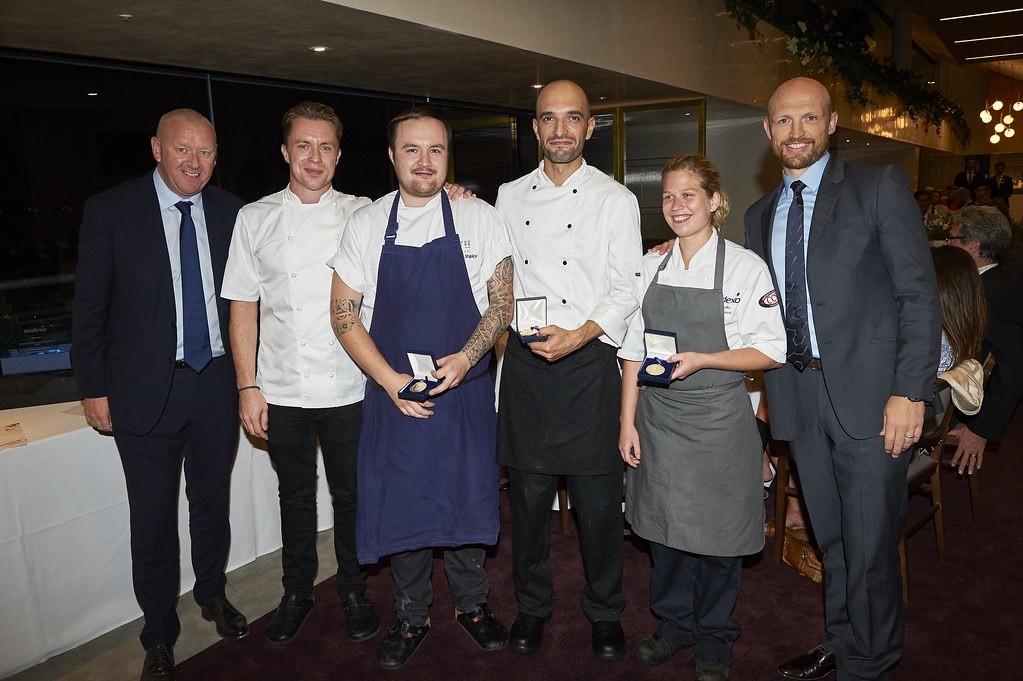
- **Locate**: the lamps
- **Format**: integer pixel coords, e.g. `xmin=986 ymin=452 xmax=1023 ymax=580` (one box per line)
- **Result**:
xmin=979 ymin=90 xmax=1023 ymax=144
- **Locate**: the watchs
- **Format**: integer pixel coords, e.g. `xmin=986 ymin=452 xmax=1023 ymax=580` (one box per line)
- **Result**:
xmin=906 ymin=397 xmax=923 ymax=402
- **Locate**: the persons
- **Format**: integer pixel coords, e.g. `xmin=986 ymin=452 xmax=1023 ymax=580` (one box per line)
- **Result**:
xmin=744 ymin=77 xmax=943 ymax=681
xmin=219 ymin=102 xmax=475 ymax=646
xmin=69 ymin=108 xmax=248 ymax=680
xmin=326 ymin=106 xmax=513 ymax=670
xmin=619 ymin=155 xmax=786 ymax=681
xmin=746 ymin=158 xmax=1023 ymax=588
xmin=493 ymin=78 xmax=643 ymax=663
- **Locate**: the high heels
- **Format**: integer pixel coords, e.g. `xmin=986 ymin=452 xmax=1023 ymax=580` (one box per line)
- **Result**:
xmin=764 ymin=521 xmax=810 ymax=543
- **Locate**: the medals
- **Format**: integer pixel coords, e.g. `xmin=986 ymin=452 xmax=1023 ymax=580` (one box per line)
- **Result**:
xmin=410 ymin=379 xmax=427 ymax=393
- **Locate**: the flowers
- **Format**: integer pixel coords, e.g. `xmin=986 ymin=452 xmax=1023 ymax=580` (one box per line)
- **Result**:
xmin=928 ymin=215 xmax=952 ymax=241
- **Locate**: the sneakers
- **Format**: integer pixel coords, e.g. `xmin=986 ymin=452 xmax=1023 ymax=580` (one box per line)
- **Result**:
xmin=634 ymin=632 xmax=696 ymax=666
xmin=694 ymin=658 xmax=731 ymax=681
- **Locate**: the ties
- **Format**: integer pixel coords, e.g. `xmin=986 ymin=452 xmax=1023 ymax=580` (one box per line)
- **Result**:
xmin=998 ymin=177 xmax=1001 ymax=189
xmin=175 ymin=200 xmax=212 ymax=373
xmin=931 ymin=206 xmax=935 ymax=215
xmin=968 ymin=172 xmax=972 ymax=186
xmin=787 ymin=180 xmax=813 ymax=373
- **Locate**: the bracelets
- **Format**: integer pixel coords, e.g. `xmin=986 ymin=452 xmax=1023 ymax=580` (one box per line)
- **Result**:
xmin=238 ymin=386 xmax=260 ymax=392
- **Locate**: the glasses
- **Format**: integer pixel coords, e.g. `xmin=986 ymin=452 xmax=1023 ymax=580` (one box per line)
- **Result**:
xmin=945 ymin=235 xmax=974 ymax=246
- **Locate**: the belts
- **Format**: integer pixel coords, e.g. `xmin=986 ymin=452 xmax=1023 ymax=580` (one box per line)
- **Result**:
xmin=175 ymin=360 xmax=191 ymax=369
xmin=806 ymin=359 xmax=822 ymax=371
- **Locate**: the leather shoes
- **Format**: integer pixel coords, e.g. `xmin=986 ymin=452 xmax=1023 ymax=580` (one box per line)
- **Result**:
xmin=140 ymin=643 xmax=177 ymax=681
xmin=266 ymin=593 xmax=316 ymax=645
xmin=376 ymin=616 xmax=431 ymax=669
xmin=776 ymin=644 xmax=835 ymax=681
xmin=201 ymin=597 xmax=249 ymax=640
xmin=341 ymin=592 xmax=380 ymax=642
xmin=509 ymin=611 xmax=548 ymax=654
xmin=592 ymin=620 xmax=627 ymax=663
xmin=454 ymin=603 xmax=509 ymax=651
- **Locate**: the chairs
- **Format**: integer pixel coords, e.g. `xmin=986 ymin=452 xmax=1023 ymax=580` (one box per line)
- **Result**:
xmin=772 ymin=353 xmax=995 ymax=607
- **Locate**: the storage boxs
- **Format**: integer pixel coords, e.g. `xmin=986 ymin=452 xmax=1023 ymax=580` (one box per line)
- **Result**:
xmin=637 ymin=329 xmax=678 ymax=386
xmin=398 ymin=349 xmax=441 ymax=406
xmin=515 ymin=297 xmax=548 ymax=344
xmin=1 ymin=342 xmax=73 ymax=376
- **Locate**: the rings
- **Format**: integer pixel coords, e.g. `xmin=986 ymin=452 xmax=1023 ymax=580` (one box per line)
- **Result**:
xmin=969 ymin=455 xmax=976 ymax=458
xmin=905 ymin=435 xmax=914 ymax=438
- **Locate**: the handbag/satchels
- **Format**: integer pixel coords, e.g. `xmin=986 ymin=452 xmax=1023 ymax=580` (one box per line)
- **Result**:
xmin=782 ymin=532 xmax=825 ymax=583
